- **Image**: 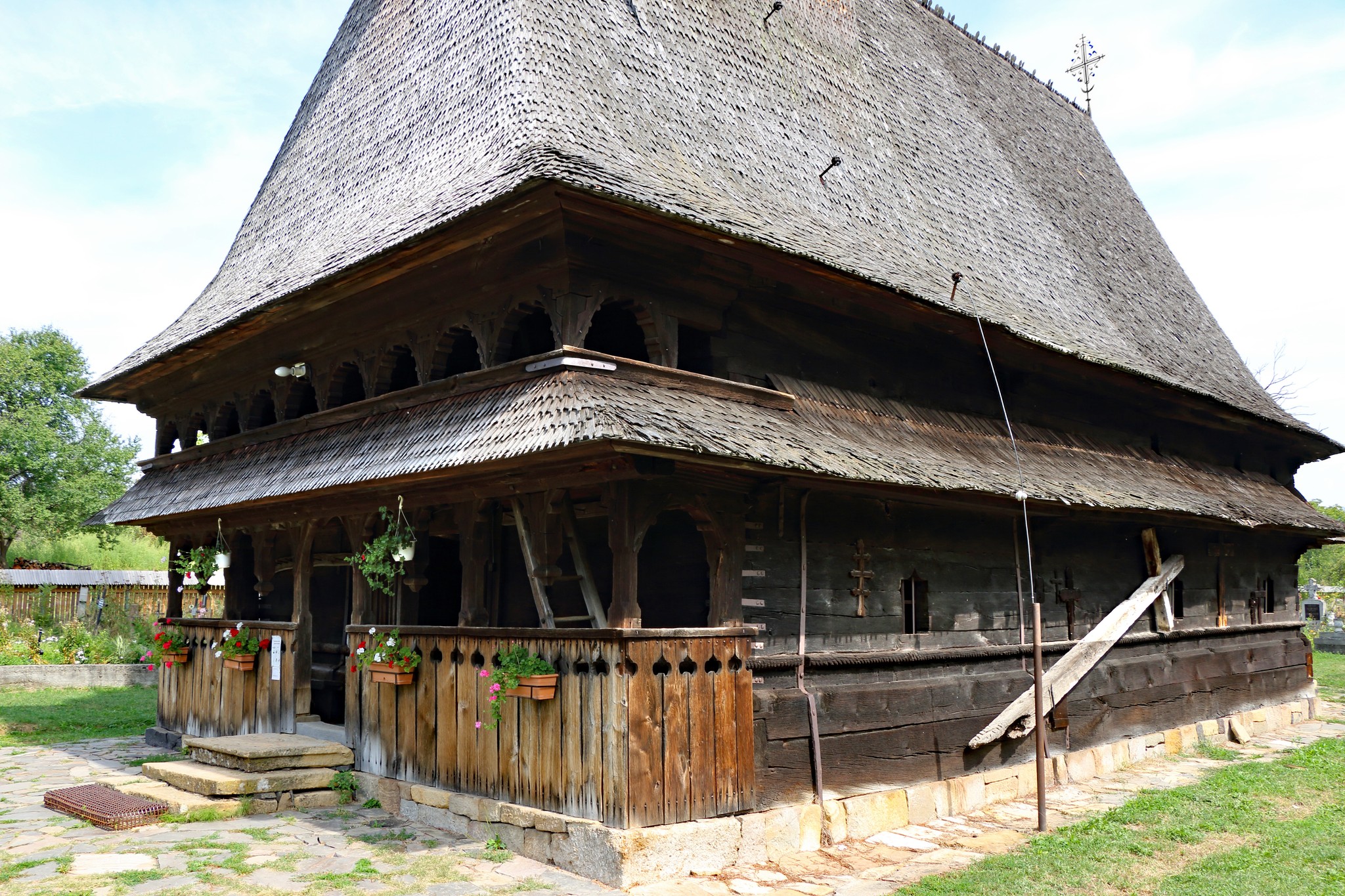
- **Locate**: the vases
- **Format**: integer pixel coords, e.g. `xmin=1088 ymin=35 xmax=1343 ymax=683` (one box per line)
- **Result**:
xmin=220 ymin=650 xmax=256 ymax=672
xmin=390 ymin=539 xmax=416 ymax=563
xmin=368 ymin=660 xmax=415 ymax=687
xmin=207 ymin=549 xmax=231 ymax=572
xmin=161 ymin=645 xmax=189 ymax=666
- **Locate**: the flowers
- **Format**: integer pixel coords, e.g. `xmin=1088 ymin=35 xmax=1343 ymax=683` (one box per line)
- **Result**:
xmin=154 ymin=541 xmax=230 ymax=595
xmin=208 ymin=622 xmax=271 ymax=661
xmin=347 ymin=626 xmax=422 ymax=676
xmin=138 ymin=617 xmax=189 ymax=673
xmin=500 ymin=671 xmax=559 ymax=704
xmin=473 ymin=638 xmax=556 ymax=732
xmin=341 ymin=505 xmax=416 ymax=598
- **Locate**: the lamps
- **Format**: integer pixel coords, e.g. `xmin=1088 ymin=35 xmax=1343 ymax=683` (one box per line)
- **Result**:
xmin=272 ymin=360 xmax=307 ymax=379
